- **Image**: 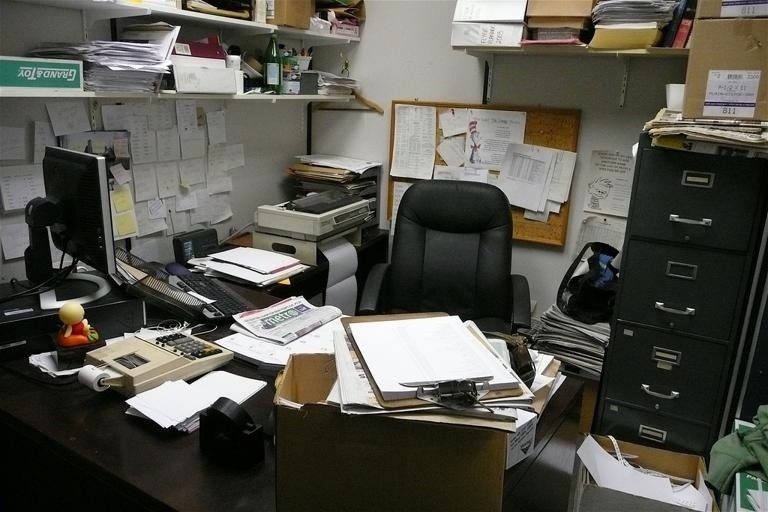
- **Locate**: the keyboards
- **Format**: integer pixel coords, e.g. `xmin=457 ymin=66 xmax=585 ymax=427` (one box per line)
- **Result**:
xmin=169 ymin=275 xmax=259 ymax=322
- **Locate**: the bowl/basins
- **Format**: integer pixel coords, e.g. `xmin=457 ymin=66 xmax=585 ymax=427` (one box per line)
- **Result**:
xmin=240 ymin=60 xmax=263 ymax=80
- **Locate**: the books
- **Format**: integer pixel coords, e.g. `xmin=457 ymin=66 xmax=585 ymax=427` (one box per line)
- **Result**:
xmin=181 ymin=0 xmax=251 ymax=20
xmin=214 ymin=315 xmax=352 ymax=369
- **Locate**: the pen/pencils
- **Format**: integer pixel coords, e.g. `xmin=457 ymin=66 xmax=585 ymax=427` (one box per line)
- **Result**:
xmin=142 ymin=300 xmax=147 ymax=328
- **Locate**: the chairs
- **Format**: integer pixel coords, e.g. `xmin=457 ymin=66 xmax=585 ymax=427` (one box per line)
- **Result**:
xmin=351 ymin=176 xmax=531 ymax=337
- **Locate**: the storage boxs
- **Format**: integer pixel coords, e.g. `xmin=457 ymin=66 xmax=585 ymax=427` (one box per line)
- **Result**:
xmin=267 ymin=349 xmax=510 ymax=510
xmin=677 ymin=16 xmax=766 ymax=121
xmin=693 ymin=1 xmax=766 ymax=19
xmin=569 ymin=431 xmax=720 ymax=512
xmin=449 ymin=0 xmax=598 ymax=49
xmin=264 ymin=0 xmax=312 ymax=29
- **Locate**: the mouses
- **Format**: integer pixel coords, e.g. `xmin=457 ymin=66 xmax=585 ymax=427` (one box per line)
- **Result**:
xmin=166 ymin=263 xmax=191 ymax=274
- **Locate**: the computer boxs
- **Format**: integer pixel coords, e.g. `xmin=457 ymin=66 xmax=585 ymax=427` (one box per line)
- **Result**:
xmin=0 ymin=270 xmax=141 ymax=365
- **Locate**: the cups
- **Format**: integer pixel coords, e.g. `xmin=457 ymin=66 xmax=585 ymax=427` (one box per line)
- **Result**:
xmin=225 ymin=54 xmax=242 ymax=69
xmin=664 ymin=84 xmax=685 ymax=109
xmin=247 ymin=57 xmax=263 ymax=73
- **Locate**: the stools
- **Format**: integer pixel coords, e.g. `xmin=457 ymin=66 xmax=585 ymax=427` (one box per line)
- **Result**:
xmin=558 ymin=363 xmax=600 ymax=437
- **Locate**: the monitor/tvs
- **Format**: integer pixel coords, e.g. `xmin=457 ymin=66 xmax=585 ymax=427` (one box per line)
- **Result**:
xmin=23 ymin=145 xmax=117 ymax=311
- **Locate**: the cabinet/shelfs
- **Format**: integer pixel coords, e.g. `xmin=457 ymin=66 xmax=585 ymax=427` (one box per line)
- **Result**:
xmin=585 ymin=133 xmax=767 ymax=470
xmin=0 ymin=1 xmax=361 ymax=104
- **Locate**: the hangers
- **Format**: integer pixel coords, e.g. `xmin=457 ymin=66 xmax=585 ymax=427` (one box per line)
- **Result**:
xmin=309 ymin=66 xmax=385 ymax=116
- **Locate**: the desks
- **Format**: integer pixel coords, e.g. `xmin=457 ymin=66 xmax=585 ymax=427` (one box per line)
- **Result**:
xmin=219 ymin=219 xmax=389 ymax=295
xmin=0 ymin=275 xmax=586 ymax=512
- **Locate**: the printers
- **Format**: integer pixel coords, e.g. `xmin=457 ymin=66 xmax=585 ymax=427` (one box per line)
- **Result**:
xmin=252 ymin=189 xmax=375 ymax=267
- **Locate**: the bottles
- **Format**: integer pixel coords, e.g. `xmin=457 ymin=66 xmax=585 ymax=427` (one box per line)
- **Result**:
xmin=263 ymin=33 xmax=282 ymax=94
